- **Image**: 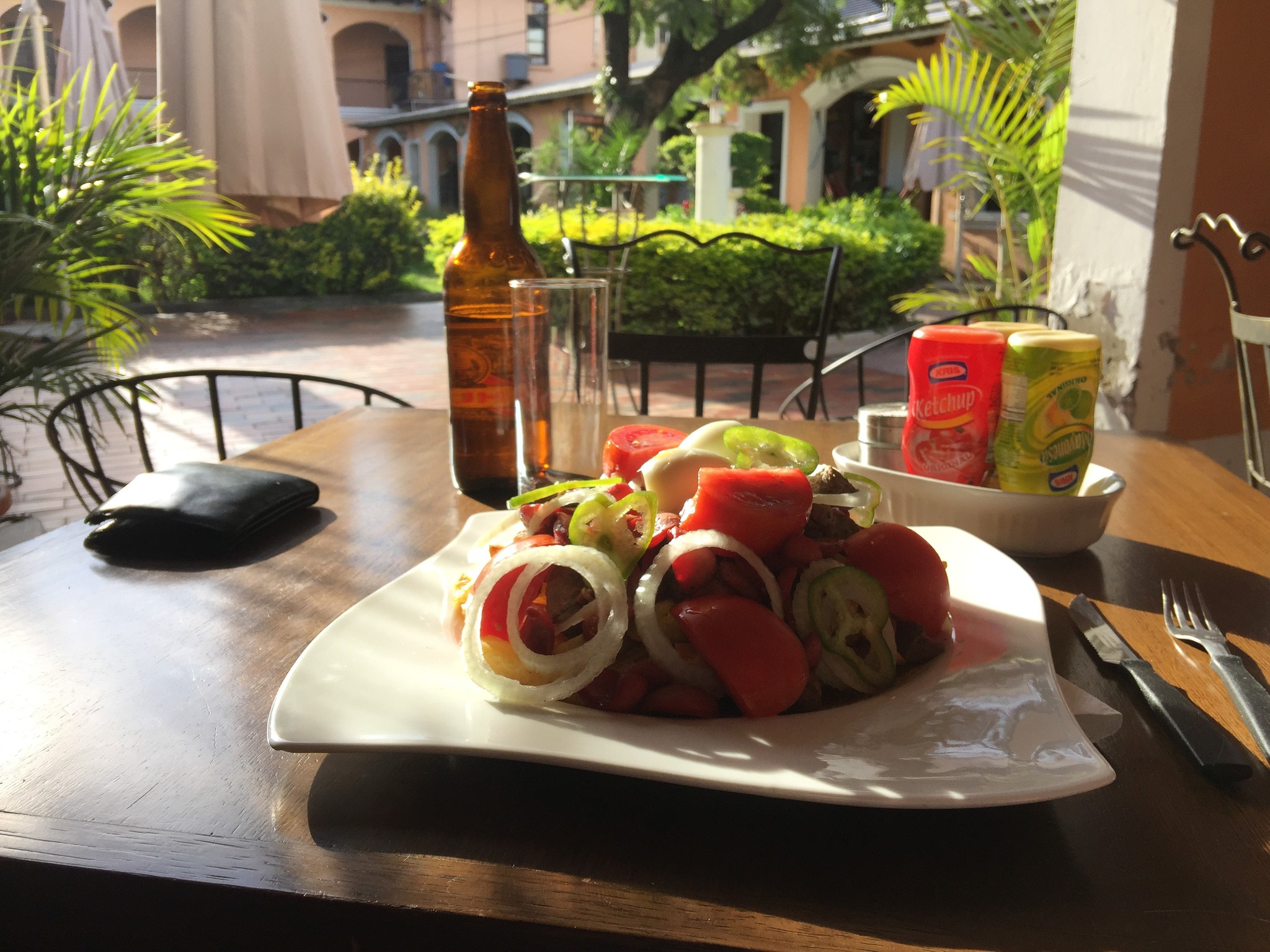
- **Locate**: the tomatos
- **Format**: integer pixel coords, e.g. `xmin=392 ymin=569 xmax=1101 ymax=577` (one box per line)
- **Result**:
xmin=451 ymin=420 xmax=950 ymax=724
xmin=915 ymin=423 xmax=972 ymax=465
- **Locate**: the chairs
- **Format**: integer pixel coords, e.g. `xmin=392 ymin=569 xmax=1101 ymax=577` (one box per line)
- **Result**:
xmin=46 ymin=371 xmax=411 ymax=508
xmin=1169 ymin=212 xmax=1269 ymax=496
xmin=567 ymin=232 xmax=844 ymax=423
xmin=780 ymin=301 xmax=1069 ymax=423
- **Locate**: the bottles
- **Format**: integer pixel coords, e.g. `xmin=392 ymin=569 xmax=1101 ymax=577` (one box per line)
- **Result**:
xmin=853 ymin=399 xmax=913 ymax=478
xmin=901 ymin=320 xmax=1003 ymax=491
xmin=969 ymin=320 xmax=1047 ymax=474
xmin=443 ymin=79 xmax=551 ymax=499
xmin=997 ymin=328 xmax=1102 ymax=496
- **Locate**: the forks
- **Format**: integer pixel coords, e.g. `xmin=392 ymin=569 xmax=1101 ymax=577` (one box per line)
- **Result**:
xmin=1160 ymin=576 xmax=1270 ymax=773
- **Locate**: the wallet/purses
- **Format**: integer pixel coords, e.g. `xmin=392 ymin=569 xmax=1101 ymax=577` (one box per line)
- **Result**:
xmin=84 ymin=462 xmax=319 ymax=562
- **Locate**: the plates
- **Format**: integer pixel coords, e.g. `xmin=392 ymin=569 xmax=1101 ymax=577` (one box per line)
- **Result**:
xmin=266 ymin=472 xmax=1120 ymax=820
xmin=833 ymin=432 xmax=1128 ymax=559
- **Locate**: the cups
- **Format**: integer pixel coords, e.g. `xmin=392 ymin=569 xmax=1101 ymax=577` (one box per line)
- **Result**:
xmin=509 ymin=277 xmax=611 ymax=493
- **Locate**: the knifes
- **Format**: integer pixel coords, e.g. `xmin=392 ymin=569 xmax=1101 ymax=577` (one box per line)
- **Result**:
xmin=1068 ymin=592 xmax=1257 ymax=784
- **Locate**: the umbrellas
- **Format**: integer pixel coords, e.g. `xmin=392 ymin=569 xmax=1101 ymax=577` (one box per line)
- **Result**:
xmin=154 ymin=0 xmax=356 ymax=232
xmin=47 ymin=0 xmax=144 ymax=174
xmin=899 ymin=0 xmax=998 ymax=299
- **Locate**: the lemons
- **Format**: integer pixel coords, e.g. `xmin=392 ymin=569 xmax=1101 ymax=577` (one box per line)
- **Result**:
xmin=1047 ymin=384 xmax=1095 ymax=428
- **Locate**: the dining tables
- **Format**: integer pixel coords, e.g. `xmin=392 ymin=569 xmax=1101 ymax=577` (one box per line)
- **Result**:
xmin=0 ymin=406 xmax=1270 ymax=951
